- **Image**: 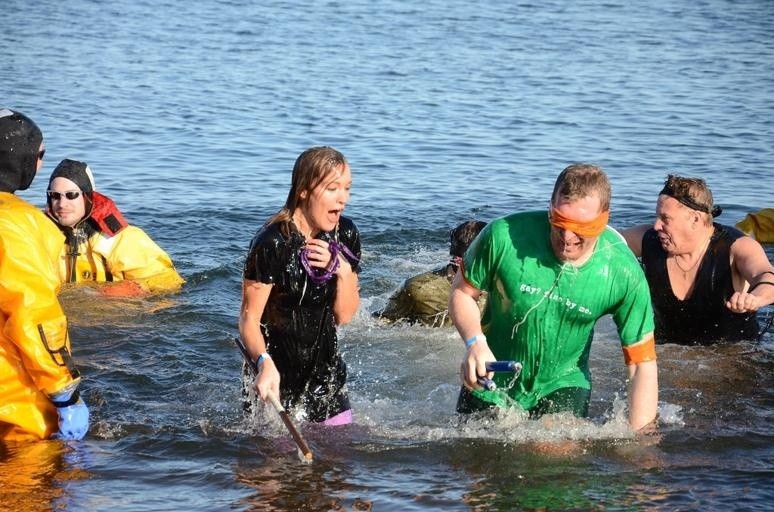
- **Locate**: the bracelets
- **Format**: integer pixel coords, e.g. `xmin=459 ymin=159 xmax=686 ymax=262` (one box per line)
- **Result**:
xmin=256 ymin=353 xmax=272 ymax=368
xmin=465 ymin=334 xmax=486 ymax=346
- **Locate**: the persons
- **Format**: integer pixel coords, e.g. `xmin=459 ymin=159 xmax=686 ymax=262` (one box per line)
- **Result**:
xmin=734 ymin=207 xmax=774 ymax=244
xmin=372 ymin=222 xmax=488 ymax=329
xmin=43 ymin=158 xmax=187 ymax=298
xmin=618 ymin=173 xmax=773 ymax=346
xmin=242 ymin=145 xmax=360 ymax=425
xmin=0 ymin=108 xmax=90 ymax=442
xmin=448 ymin=163 xmax=659 ymax=430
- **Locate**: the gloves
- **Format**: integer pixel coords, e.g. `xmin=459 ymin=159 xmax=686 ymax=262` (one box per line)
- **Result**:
xmin=48 ymin=386 xmax=91 ymax=442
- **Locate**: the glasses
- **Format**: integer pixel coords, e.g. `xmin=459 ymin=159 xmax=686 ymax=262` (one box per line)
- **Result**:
xmin=35 ymin=148 xmax=46 ymax=160
xmin=46 ymin=190 xmax=83 ymax=200
